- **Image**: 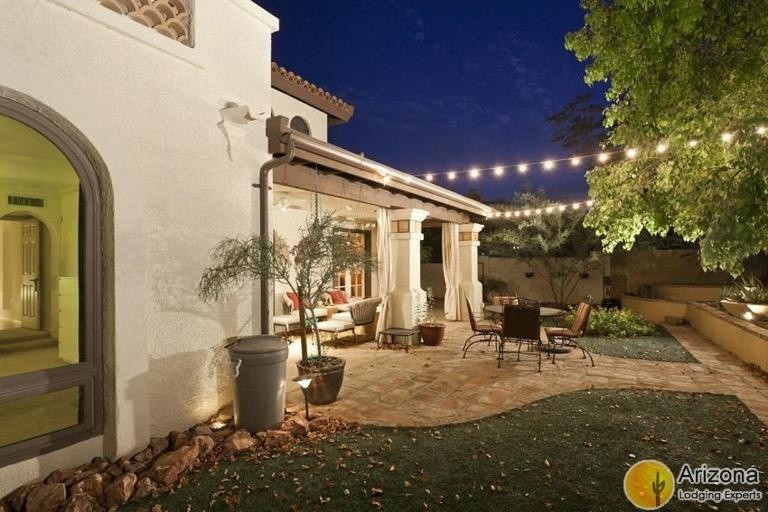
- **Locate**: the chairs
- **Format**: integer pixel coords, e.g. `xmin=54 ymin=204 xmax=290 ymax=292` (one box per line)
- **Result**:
xmin=263 ymin=287 xmax=382 ymax=344
xmin=463 ymin=296 xmax=597 ymax=373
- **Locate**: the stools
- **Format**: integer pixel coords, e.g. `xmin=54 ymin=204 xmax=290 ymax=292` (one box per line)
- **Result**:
xmin=263 ymin=315 xmax=357 ymax=350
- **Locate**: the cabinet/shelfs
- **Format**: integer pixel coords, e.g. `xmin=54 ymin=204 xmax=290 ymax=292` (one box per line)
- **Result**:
xmin=57 ymin=275 xmax=79 ymax=364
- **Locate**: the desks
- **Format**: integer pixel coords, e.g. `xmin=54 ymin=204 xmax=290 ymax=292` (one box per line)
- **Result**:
xmin=378 ymin=327 xmax=422 ymax=354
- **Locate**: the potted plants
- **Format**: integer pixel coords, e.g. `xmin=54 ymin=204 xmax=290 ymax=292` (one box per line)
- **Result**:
xmin=416 ymin=286 xmax=446 ymax=345
xmin=722 ymin=274 xmax=768 ymax=320
xmin=195 ymin=210 xmax=380 ymax=403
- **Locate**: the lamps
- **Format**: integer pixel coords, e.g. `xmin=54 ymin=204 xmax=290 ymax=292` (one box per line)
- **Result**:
xmin=293 ymin=373 xmax=313 ymax=421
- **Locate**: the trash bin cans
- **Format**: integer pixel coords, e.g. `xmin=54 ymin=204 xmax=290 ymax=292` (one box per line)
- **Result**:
xmin=224 ymin=335 xmax=288 ymax=435
